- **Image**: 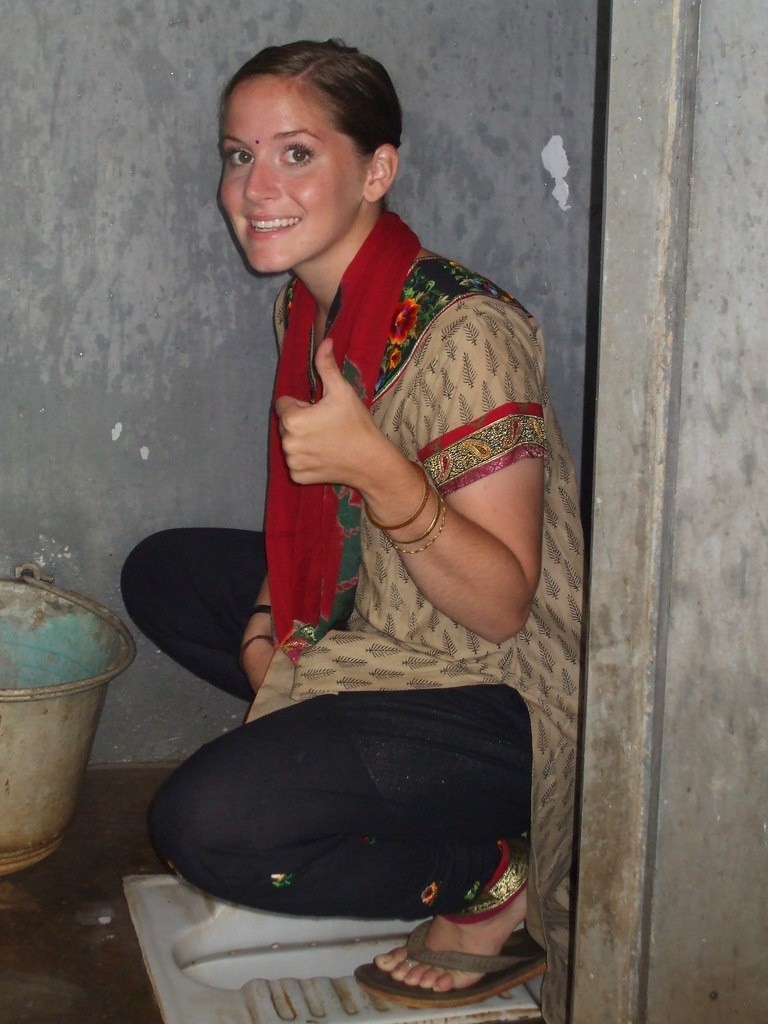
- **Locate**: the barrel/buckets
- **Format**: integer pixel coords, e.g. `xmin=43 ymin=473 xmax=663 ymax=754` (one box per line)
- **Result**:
xmin=0 ymin=564 xmax=135 ymax=877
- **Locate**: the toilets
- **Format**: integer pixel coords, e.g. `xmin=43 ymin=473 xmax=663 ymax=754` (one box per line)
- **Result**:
xmin=123 ymin=870 xmax=544 ymax=1021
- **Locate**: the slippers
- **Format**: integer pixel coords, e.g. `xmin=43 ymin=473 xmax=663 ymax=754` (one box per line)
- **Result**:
xmin=354 ymin=919 xmax=548 ymax=1009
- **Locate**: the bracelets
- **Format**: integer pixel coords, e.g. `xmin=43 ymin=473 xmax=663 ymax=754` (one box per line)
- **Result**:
xmin=365 ymin=461 xmax=429 ymax=530
xmin=249 ymin=604 xmax=271 ymax=618
xmin=390 ymin=498 xmax=446 ymax=554
xmin=238 ymin=635 xmax=273 ymax=669
xmin=382 ymin=484 xmax=441 ymax=544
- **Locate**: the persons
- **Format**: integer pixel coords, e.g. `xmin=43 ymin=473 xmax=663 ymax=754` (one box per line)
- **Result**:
xmin=121 ymin=38 xmax=582 ymax=1008
